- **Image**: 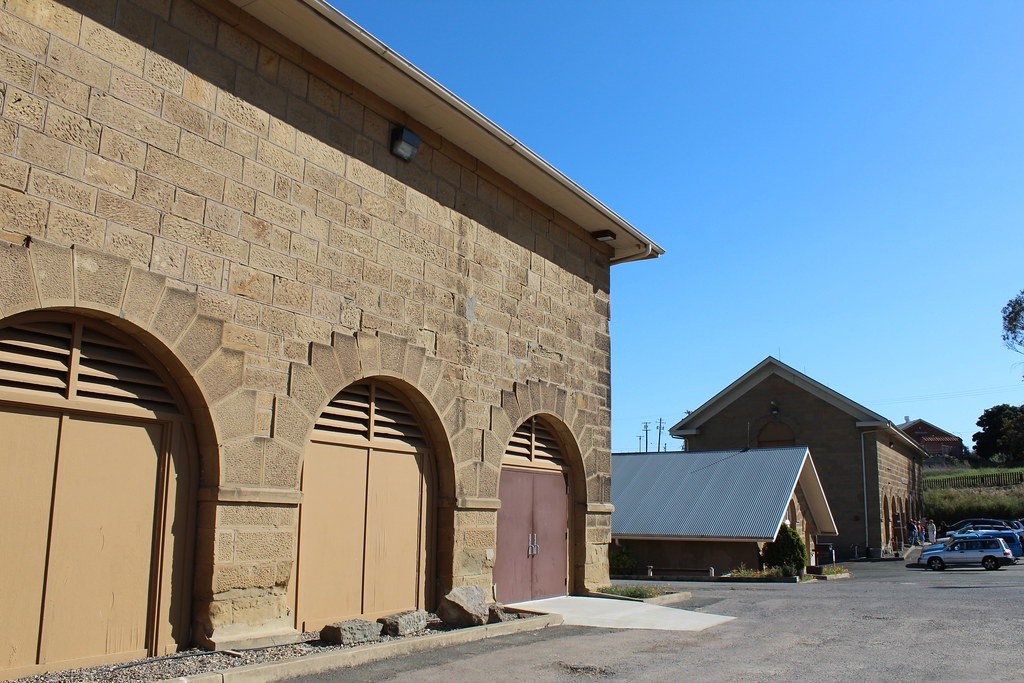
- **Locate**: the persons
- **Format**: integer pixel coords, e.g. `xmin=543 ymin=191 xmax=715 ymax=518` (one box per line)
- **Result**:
xmin=908 ymin=518 xmax=964 ymax=547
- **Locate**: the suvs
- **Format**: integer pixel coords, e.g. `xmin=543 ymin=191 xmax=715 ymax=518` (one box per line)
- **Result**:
xmin=918 ymin=537 xmax=1016 ymax=572
xmin=921 ymin=519 xmax=1024 ymax=565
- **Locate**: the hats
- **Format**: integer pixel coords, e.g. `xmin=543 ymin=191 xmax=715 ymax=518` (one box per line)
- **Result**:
xmin=917 ymin=521 xmax=921 ymax=523
xmin=910 ymin=519 xmax=913 ymax=522
